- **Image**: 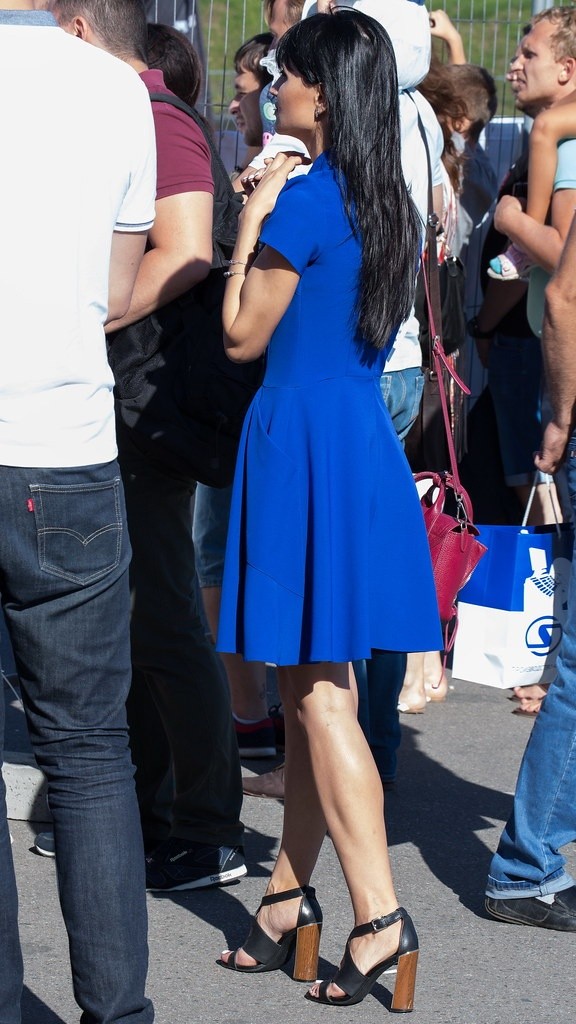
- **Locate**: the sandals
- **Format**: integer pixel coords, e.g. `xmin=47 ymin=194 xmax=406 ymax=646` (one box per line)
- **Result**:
xmin=514 ymin=699 xmax=543 ymax=717
xmin=487 ymin=244 xmax=539 ymax=281
xmin=511 ymin=682 xmax=551 ymax=702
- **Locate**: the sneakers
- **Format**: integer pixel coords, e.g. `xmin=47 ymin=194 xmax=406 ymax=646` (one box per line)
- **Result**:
xmin=232 ymin=717 xmax=277 ymax=758
xmin=268 ymin=702 xmax=285 ymax=752
xmin=144 ymin=835 xmax=248 ymax=891
xmin=34 ymin=832 xmax=55 ymax=857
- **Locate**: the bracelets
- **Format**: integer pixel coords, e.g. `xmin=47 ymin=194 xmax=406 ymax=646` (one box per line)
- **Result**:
xmin=223 ymin=271 xmax=250 ymax=280
xmin=228 ymin=258 xmax=253 ymax=267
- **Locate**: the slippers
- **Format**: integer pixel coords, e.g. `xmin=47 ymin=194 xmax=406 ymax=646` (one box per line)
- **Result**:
xmin=426 ymin=697 xmax=445 ymax=702
xmin=397 ymin=702 xmax=424 ymax=713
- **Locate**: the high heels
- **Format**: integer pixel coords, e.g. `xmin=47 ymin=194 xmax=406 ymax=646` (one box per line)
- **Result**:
xmin=220 ymin=886 xmax=323 ymax=981
xmin=306 ymin=906 xmax=420 ymax=1013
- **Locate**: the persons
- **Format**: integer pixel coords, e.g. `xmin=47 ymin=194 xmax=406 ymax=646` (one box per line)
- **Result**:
xmin=33 ymin=0 xmax=248 ymax=893
xmin=137 ymin=0 xmax=576 ymax=934
xmin=0 ymin=0 xmax=157 ymax=1024
xmin=214 ymin=10 xmax=444 ymax=1011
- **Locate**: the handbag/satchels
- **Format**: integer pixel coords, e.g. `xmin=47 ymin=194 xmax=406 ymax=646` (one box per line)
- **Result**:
xmin=424 ymin=257 xmax=467 ymax=356
xmin=414 ymin=471 xmax=488 ymax=621
xmin=404 ymin=348 xmax=468 ymax=469
xmin=106 ymin=92 xmax=269 ymax=489
xmin=451 ymin=467 xmax=575 ymax=690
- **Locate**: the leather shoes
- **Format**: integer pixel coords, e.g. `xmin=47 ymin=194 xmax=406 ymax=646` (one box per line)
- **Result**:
xmin=485 ymin=884 xmax=576 ymax=933
xmin=241 ymin=762 xmax=285 ymax=801
